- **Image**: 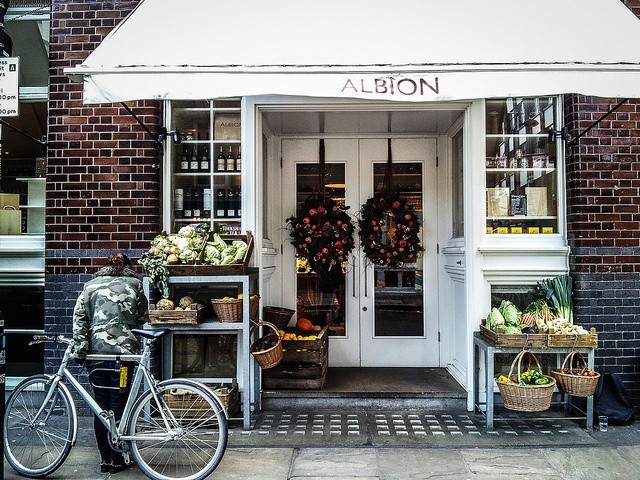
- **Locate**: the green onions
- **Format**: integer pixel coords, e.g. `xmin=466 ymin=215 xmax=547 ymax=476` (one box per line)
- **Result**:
xmin=535 ymin=273 xmax=574 ymax=325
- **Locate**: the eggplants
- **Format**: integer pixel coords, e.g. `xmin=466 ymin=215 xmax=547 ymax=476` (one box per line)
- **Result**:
xmin=521 ymin=327 xmax=536 ymax=334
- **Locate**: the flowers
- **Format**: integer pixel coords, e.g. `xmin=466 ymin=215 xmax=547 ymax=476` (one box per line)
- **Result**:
xmin=355 ymin=190 xmax=424 ymax=266
xmin=284 ymin=195 xmax=355 ymax=271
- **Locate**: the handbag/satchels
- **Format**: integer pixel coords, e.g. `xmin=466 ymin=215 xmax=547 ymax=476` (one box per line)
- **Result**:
xmin=0 ymin=206 xmax=22 ymax=235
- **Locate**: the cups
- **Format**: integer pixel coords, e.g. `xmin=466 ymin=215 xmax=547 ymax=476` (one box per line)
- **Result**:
xmin=598 ymin=416 xmax=608 ymax=432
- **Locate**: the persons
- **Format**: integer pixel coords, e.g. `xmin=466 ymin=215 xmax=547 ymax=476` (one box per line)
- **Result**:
xmin=72 ymin=251 xmax=148 ymax=474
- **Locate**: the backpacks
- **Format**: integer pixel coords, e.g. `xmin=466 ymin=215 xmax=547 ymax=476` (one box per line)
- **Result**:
xmin=576 ymin=372 xmax=637 ymax=426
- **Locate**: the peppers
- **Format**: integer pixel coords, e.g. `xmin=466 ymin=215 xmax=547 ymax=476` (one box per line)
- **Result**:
xmin=521 ymin=368 xmax=549 ymax=385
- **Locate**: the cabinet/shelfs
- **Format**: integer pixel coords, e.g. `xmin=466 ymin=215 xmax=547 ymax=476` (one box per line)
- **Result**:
xmin=444 ymin=96 xmax=557 ymax=240
xmin=14 ymin=177 xmax=46 ymax=235
xmin=165 ymin=96 xmax=277 ymax=247
xmin=163 ymin=268 xmax=261 ymax=428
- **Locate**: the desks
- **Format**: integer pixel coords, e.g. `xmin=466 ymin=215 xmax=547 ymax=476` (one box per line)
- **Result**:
xmin=472 ymin=335 xmax=594 ymax=431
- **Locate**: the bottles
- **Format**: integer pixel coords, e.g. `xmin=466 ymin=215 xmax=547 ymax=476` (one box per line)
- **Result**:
xmin=180 ymin=147 xmax=189 ymax=171
xmin=189 ymin=147 xmax=198 ymax=171
xmin=217 ymin=146 xmax=225 ymax=171
xmin=203 ymin=189 xmax=211 ymax=215
xmin=174 ymin=188 xmax=183 ymax=218
xmin=193 ymin=192 xmax=201 ymax=217
xmin=184 ymin=192 xmax=192 ymax=217
xmin=218 ymin=226 xmax=241 ymax=235
xmin=226 ymin=146 xmax=234 ymax=171
xmin=199 ymin=147 xmax=209 ymax=171
xmin=227 ymin=192 xmax=235 ymax=217
xmin=237 ymin=193 xmax=242 ymax=217
xmin=236 ymin=146 xmax=241 ymax=171
xmin=216 ymin=192 xmax=225 ymax=217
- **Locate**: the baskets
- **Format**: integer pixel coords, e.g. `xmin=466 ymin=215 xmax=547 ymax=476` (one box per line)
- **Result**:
xmin=210 ymin=299 xmax=259 ymax=323
xmin=551 ymin=351 xmax=601 ymax=397
xmin=263 ymin=306 xmax=295 ymax=330
xmin=495 ymin=350 xmax=556 ymax=412
xmin=249 ymin=322 xmax=283 ymax=369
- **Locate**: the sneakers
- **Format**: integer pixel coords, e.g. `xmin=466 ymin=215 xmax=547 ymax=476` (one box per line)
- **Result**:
xmin=100 ymin=460 xmax=111 ymax=472
xmin=111 ymin=459 xmax=134 ymax=474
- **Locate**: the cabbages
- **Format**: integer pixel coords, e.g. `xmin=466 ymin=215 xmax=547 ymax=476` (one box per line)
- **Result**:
xmin=151 ymin=225 xmax=247 ymax=266
xmin=487 ymin=301 xmax=528 ymax=335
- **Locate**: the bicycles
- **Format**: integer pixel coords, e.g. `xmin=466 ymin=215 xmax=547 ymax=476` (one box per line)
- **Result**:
xmin=4 ymin=329 xmax=229 ymax=480
